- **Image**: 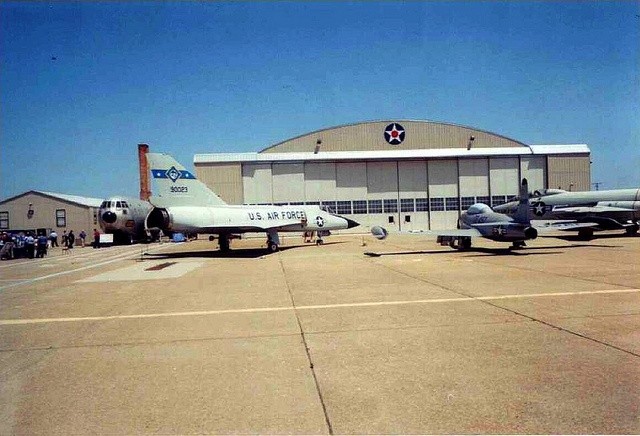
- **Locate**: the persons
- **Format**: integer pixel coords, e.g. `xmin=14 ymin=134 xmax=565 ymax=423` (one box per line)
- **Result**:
xmin=61 ymin=230 xmax=75 ymax=249
xmin=1 ymin=232 xmax=14 ymax=259
xmin=79 ymin=229 xmax=87 ymax=248
xmin=93 ymin=229 xmax=100 ymax=249
xmin=25 ymin=231 xmax=47 ymax=258
xmin=49 ymin=230 xmax=59 ymax=247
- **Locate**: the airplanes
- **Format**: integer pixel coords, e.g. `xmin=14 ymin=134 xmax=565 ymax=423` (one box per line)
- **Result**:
xmin=98 ymin=197 xmax=152 ymax=242
xmin=437 ymin=179 xmax=537 ymax=248
xmin=145 ymin=152 xmax=360 ymax=252
xmin=492 ymin=190 xmax=637 ymax=237
